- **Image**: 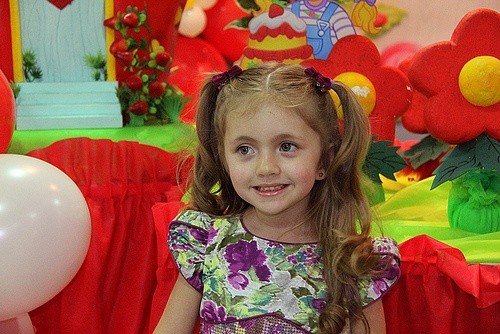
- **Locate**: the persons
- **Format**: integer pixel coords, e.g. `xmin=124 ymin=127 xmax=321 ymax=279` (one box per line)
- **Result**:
xmin=155 ymin=62 xmax=403 ymax=334
